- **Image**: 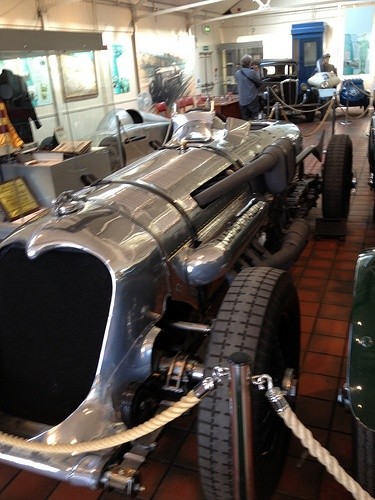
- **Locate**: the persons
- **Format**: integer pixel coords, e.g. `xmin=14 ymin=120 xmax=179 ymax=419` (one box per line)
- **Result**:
xmin=310 ymin=53 xmax=336 ymax=75
xmin=234 ymin=53 xmax=263 ymax=121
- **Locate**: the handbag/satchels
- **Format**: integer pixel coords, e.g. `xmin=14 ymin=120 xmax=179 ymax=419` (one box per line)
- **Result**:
xmin=254 ymin=81 xmax=261 ymax=88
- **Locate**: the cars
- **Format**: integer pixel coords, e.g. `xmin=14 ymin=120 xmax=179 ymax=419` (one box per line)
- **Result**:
xmin=253 ymin=55 xmax=317 ymax=124
